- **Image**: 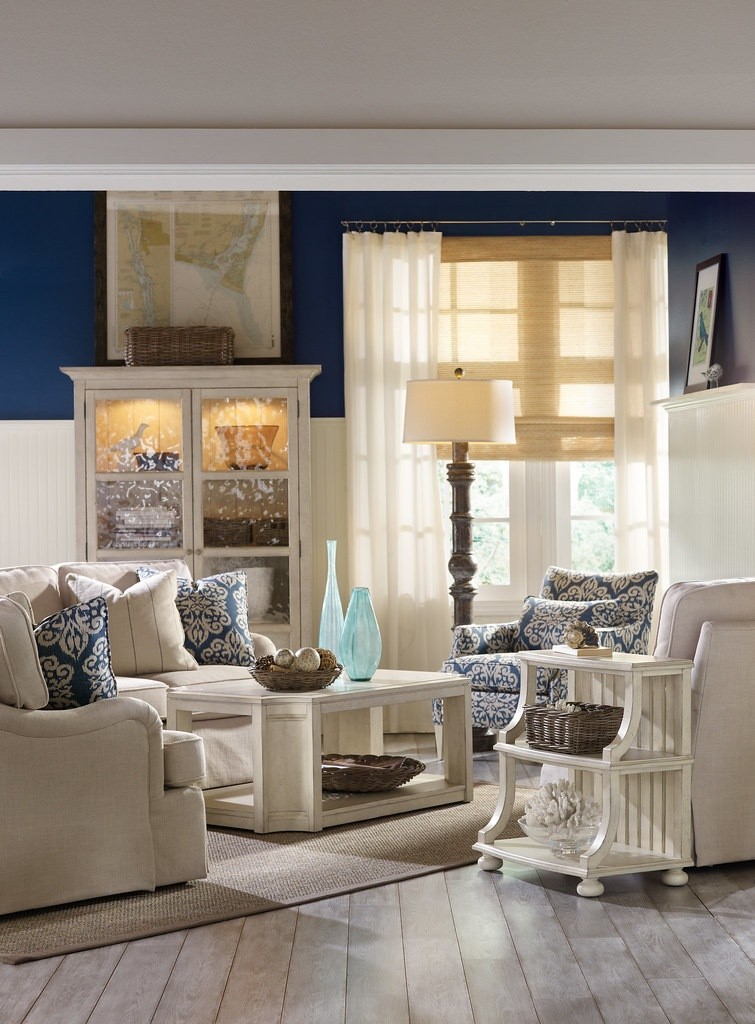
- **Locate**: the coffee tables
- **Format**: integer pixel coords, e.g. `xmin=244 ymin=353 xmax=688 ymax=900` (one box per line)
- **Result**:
xmin=166 ymin=669 xmax=474 ymax=833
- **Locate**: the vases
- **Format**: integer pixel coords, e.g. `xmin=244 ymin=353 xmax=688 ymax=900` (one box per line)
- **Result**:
xmin=319 ymin=539 xmax=343 ymax=664
xmin=236 ymin=567 xmax=274 ymax=623
xmin=339 ymin=588 xmax=383 ymax=681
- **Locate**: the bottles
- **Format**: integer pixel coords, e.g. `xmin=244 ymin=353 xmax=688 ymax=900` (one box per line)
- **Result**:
xmin=318 ymin=539 xmax=345 ymax=679
xmin=337 ymin=586 xmax=382 ymax=683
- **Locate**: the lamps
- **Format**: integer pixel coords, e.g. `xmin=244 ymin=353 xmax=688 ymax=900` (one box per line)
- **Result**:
xmin=403 ymin=368 xmax=518 ymax=751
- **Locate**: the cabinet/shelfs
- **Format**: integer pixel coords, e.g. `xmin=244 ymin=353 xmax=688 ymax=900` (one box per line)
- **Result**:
xmin=473 ymin=651 xmax=696 ymax=897
xmin=59 ymin=364 xmax=322 ymax=650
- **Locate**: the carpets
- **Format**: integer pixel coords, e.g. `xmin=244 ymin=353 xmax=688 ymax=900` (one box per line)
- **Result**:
xmin=1 ymin=780 xmax=541 ymax=966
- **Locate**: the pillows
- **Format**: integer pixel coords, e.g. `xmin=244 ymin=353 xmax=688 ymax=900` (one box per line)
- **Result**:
xmin=136 ymin=566 xmax=257 ymax=667
xmin=65 ymin=569 xmax=199 ymax=676
xmin=511 ymin=596 xmax=623 ymax=653
xmin=30 ymin=596 xmax=118 ymax=709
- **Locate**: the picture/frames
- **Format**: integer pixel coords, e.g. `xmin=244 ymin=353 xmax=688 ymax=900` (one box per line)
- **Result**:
xmin=684 ymin=252 xmax=728 ymax=394
xmin=93 ymin=191 xmax=296 ymax=366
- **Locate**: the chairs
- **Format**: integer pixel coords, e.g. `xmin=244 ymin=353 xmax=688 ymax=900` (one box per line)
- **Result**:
xmin=431 ymin=566 xmax=659 ymax=762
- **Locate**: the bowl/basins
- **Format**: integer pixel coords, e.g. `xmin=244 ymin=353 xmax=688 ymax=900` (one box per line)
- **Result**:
xmin=133 ymin=451 xmax=180 ymax=471
xmin=518 ymin=817 xmax=600 ymax=861
xmin=214 ymin=424 xmax=279 ymax=470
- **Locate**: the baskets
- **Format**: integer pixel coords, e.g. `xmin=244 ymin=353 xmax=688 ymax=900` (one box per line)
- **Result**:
xmin=321 ymin=751 xmax=426 ymax=792
xmin=251 ymin=518 xmax=289 ymax=546
xmin=247 ymin=657 xmax=342 ymax=692
xmin=125 ymin=326 xmax=235 ymax=366
xmin=203 ymin=518 xmax=248 ymax=546
xmin=522 ymin=699 xmax=625 ymax=756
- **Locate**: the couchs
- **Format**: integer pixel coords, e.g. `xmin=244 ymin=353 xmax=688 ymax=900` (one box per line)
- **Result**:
xmin=1 ymin=559 xmax=277 ymax=914
xmin=652 ymin=577 xmax=755 ymax=867
